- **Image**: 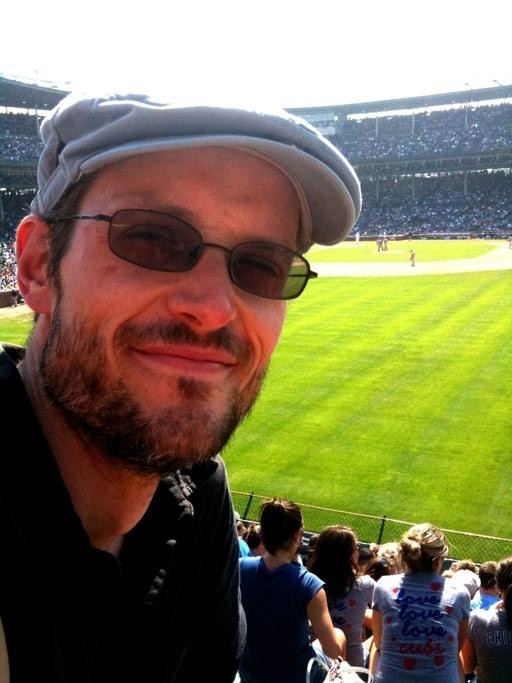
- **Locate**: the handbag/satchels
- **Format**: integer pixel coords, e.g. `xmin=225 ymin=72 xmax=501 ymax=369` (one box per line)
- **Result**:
xmin=306 ymin=655 xmax=375 ymax=683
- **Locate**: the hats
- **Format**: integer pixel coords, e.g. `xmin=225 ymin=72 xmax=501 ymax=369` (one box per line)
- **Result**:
xmin=30 ymin=91 xmax=362 ymax=256
xmin=453 ymin=569 xmax=481 ymax=596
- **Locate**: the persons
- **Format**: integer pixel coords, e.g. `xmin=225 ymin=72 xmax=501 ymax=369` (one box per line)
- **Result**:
xmin=0 ymin=111 xmax=44 ymax=309
xmin=322 ymin=101 xmax=512 ymax=252
xmin=235 ymin=496 xmax=512 ymax=682
xmin=1 ymin=85 xmax=362 ymax=682
xmin=410 ymin=250 xmax=416 ymax=267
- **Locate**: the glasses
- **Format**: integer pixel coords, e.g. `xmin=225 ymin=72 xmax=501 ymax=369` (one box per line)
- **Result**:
xmin=48 ymin=210 xmax=317 ymax=300
